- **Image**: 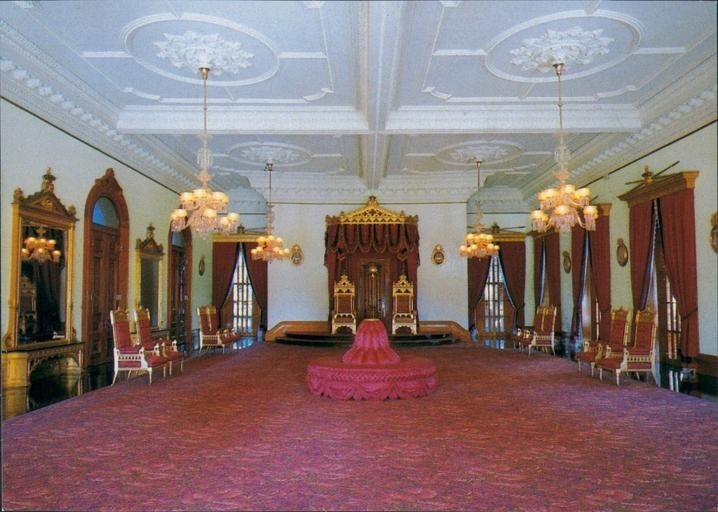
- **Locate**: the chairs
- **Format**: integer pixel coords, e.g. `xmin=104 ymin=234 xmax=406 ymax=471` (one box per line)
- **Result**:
xmin=597 ymin=302 xmax=659 ymax=385
xmin=110 ymin=307 xmax=167 ymax=385
xmin=197 ymin=303 xmax=240 ymax=354
xmin=392 ymin=274 xmax=417 ymax=336
xmin=332 ymin=274 xmax=357 ymax=334
xmin=576 ymin=306 xmax=630 ymax=376
xmin=513 ymin=304 xmax=557 ymax=357
xmin=134 ymin=306 xmax=185 ymax=376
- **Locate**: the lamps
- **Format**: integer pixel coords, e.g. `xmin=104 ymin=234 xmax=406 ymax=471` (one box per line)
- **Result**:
xmin=21 ymin=223 xmax=61 ymax=264
xmin=458 ymin=161 xmax=500 ymax=258
xmin=531 ymin=63 xmax=598 ymax=231
xmin=251 ymin=159 xmax=290 ymax=262
xmin=171 ymin=68 xmax=239 ymax=235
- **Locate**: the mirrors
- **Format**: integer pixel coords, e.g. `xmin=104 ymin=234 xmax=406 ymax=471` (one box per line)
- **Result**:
xmin=137 ymin=224 xmax=165 ymax=330
xmin=9 ymin=169 xmax=80 ymax=346
